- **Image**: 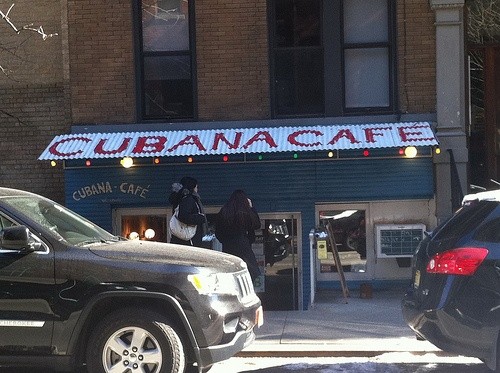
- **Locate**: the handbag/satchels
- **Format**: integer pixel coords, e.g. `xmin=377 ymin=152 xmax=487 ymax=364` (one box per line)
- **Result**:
xmin=170 ymin=195 xmax=200 ymax=241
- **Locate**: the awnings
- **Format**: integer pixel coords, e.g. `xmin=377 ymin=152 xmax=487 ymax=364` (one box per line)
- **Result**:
xmin=37 ymin=119 xmax=438 ymax=161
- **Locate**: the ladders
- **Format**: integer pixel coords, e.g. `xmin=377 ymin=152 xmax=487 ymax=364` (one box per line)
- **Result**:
xmin=325 ymin=221 xmax=350 ymax=305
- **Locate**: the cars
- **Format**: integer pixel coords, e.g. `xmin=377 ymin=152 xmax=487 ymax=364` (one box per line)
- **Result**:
xmin=402 ymin=185 xmax=500 ymax=373
xmin=0 ymin=186 xmax=265 ymax=373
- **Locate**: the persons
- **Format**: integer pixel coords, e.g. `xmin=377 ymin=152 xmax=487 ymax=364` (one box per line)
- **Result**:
xmin=168 ymin=177 xmax=209 ymax=249
xmin=215 ymin=189 xmax=261 ymax=289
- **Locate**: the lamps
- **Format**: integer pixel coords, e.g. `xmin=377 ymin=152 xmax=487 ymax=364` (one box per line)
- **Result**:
xmin=140 ymin=228 xmax=155 ymax=239
xmin=122 ymin=157 xmax=133 ymax=169
xmin=404 ymin=146 xmax=418 ymax=158
